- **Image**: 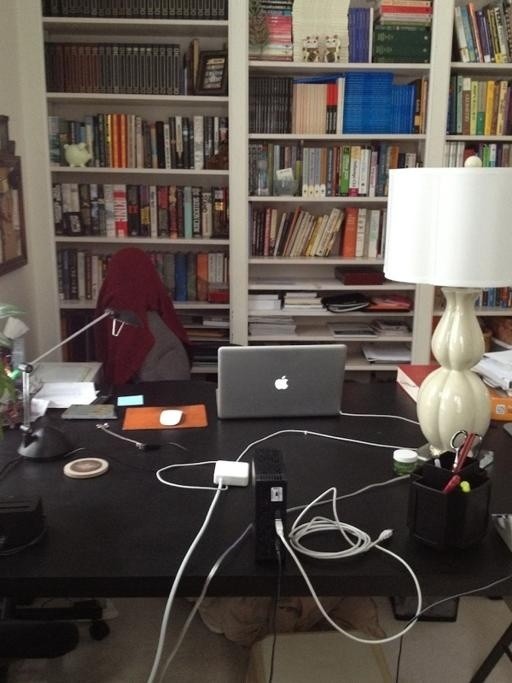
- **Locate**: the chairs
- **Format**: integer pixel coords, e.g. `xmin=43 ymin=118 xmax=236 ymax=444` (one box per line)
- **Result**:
xmin=95 ymin=248 xmax=191 ymax=383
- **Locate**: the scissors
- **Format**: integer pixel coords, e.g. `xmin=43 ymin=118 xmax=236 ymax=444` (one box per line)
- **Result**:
xmin=449 ymin=429 xmax=482 ymax=473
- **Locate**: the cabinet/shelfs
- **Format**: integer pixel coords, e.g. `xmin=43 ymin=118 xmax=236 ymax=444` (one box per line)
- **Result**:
xmin=17 ymin=1 xmax=512 ymax=378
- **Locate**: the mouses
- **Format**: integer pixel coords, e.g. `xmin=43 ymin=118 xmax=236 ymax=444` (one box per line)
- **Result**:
xmin=160 ymin=410 xmax=183 ymax=425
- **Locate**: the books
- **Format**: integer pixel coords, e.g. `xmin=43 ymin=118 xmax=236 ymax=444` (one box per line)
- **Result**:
xmin=476 ymin=286 xmax=511 ymax=308
xmin=43 ymin=1 xmax=228 ymax=366
xmin=249 ymin=0 xmax=432 ymax=364
xmin=443 ymin=1 xmax=511 ymax=167
xmin=396 ymin=350 xmax=512 ymax=423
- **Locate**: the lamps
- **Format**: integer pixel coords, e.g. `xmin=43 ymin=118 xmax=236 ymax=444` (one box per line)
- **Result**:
xmin=20 ymin=307 xmax=145 ymax=463
xmin=388 ymin=168 xmax=512 ymax=473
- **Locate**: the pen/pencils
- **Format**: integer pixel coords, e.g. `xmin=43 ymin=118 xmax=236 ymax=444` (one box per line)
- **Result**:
xmin=432 ymin=455 xmax=441 ymax=468
xmin=459 ymin=481 xmax=472 ymax=493
xmin=440 ymin=472 xmax=461 ymax=495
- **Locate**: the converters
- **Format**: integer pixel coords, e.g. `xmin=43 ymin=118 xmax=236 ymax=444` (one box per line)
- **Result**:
xmin=213 ymin=460 xmax=249 ymax=487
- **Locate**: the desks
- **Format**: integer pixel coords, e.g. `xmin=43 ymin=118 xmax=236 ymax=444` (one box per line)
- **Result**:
xmin=0 ymin=403 xmax=510 ymax=598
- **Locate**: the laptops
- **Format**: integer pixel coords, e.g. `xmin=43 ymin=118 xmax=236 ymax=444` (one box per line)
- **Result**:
xmin=215 ymin=344 xmax=347 ymax=418
